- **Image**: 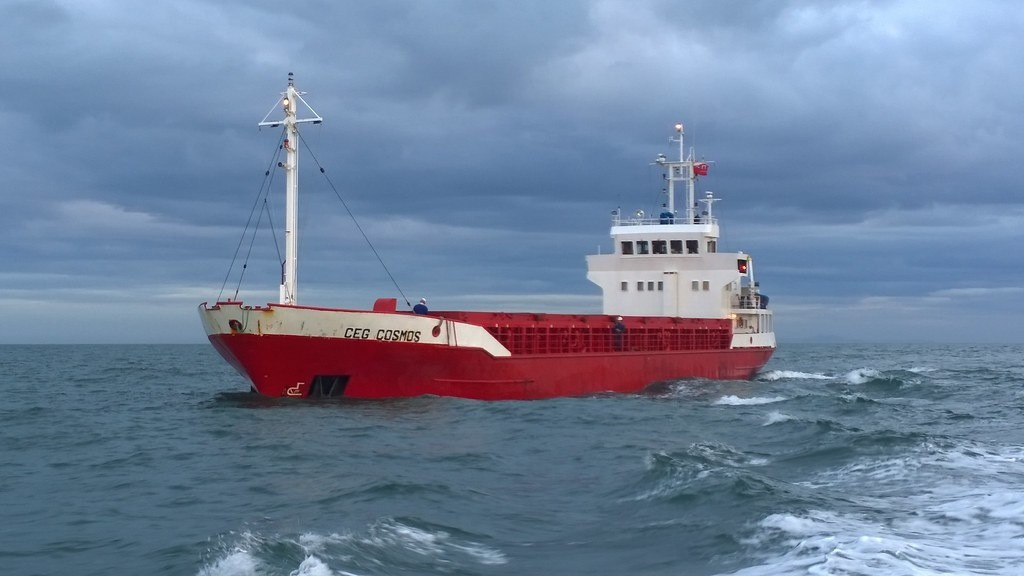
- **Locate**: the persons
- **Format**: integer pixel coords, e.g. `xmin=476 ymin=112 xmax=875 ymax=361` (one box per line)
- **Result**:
xmin=612 ymin=317 xmax=626 ymax=352
xmin=414 ymin=297 xmax=429 ymax=316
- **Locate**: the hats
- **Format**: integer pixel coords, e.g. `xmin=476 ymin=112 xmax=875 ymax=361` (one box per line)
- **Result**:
xmin=617 ymin=317 xmax=623 ymax=321
xmin=421 ymin=298 xmax=425 ymax=302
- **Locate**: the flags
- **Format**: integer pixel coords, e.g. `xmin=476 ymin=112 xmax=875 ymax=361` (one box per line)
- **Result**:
xmin=693 ymin=165 xmax=708 ymax=177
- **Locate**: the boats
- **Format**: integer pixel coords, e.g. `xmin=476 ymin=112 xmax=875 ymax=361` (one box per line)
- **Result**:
xmin=197 ymin=71 xmax=776 ymax=403
